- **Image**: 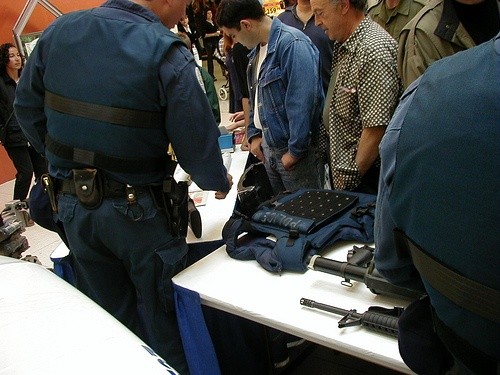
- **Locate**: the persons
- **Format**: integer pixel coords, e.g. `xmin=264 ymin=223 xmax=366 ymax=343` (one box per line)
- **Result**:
xmin=278 ymin=1 xmax=336 ymax=98
xmin=11 ymin=1 xmax=234 ymax=375
xmin=0 ymin=43 xmax=48 ymax=203
xmin=396 ymin=0 xmax=500 ymax=91
xmin=368 ymin=0 xmax=425 ymax=43
xmin=215 ymin=0 xmax=324 ymax=196
xmin=175 ymin=0 xmax=252 ymax=152
xmin=373 ymin=31 xmax=500 ymax=375
xmin=308 ymin=0 xmax=401 ymax=196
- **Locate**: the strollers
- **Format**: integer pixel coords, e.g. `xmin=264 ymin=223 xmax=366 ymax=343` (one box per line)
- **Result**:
xmin=210 ymin=32 xmax=230 ymax=101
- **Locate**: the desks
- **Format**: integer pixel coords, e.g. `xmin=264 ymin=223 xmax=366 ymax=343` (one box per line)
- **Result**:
xmin=172 ymin=230 xmax=418 ymax=375
xmin=50 ymin=143 xmax=249 ymax=286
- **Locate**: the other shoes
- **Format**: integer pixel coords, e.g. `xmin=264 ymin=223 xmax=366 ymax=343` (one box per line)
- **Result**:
xmin=212 ymin=75 xmax=217 ymax=81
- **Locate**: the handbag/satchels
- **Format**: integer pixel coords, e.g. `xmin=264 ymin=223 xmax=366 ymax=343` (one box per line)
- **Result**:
xmin=398 ymin=294 xmax=454 ymax=375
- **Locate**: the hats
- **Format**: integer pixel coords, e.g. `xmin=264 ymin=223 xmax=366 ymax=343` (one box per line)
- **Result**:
xmin=29 ymin=177 xmax=70 ymax=250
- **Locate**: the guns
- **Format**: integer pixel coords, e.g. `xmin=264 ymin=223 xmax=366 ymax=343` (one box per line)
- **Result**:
xmin=162 ymin=173 xmax=189 ymax=240
xmin=306 ymin=244 xmax=427 ymax=302
xmin=300 ymin=296 xmax=406 ymax=338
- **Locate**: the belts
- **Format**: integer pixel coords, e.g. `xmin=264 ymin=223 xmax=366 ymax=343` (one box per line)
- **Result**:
xmin=52 ymin=177 xmax=150 ymax=196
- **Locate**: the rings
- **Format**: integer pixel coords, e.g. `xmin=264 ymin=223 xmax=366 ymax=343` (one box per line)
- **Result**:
xmin=254 ymin=155 xmax=257 ymax=157
